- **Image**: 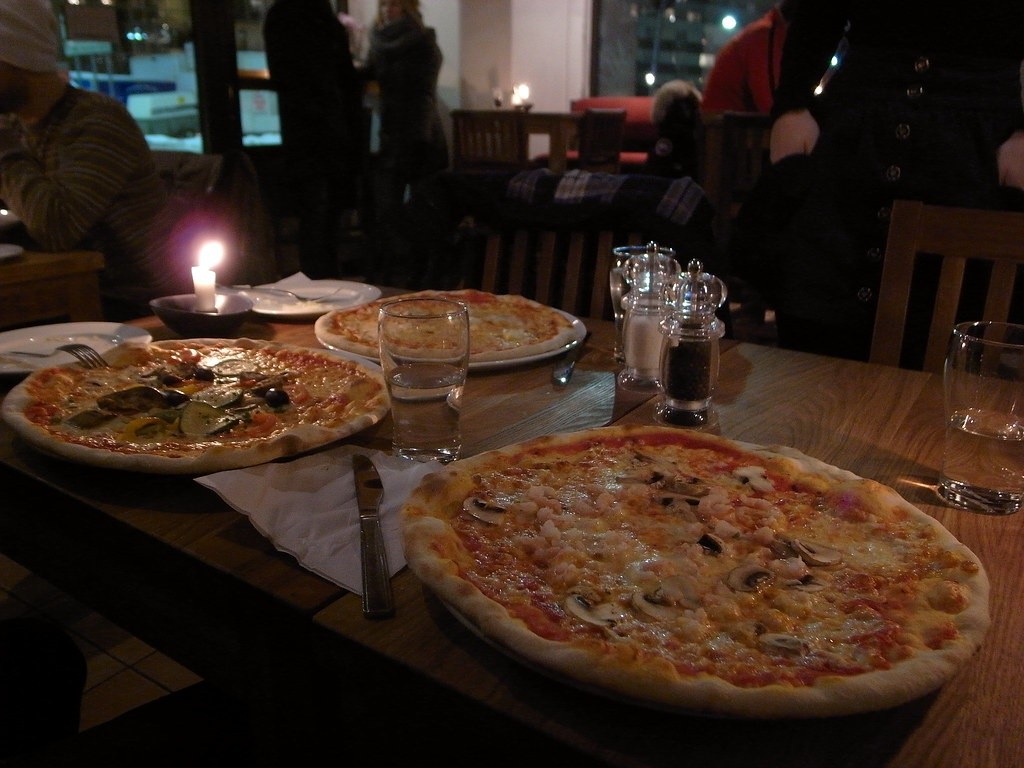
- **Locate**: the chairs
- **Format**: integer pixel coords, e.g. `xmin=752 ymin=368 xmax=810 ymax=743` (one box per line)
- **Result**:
xmin=0 ymin=554 xmax=205 ymax=767
xmin=445 ymin=106 xmax=1024 ymax=376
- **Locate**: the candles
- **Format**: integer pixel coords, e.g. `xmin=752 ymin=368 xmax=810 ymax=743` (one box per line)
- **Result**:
xmin=191 ymin=266 xmax=214 ymax=312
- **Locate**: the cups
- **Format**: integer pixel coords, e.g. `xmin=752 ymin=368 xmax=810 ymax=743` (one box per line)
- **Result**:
xmin=936 ymin=320 xmax=1024 ymax=517
xmin=377 ymin=295 xmax=469 ymax=466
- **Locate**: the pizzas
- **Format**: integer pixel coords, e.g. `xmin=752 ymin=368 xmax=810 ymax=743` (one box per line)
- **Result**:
xmin=0 ymin=337 xmax=390 ymax=473
xmin=397 ymin=421 xmax=991 ymax=713
xmin=315 ymin=288 xmax=579 ymax=364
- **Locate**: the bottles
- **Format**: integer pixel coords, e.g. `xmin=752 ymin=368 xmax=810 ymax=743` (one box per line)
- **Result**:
xmin=651 ymin=259 xmax=726 ymax=433
xmin=617 ymin=242 xmax=681 ymax=396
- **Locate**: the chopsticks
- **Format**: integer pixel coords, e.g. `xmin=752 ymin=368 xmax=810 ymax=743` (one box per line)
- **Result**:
xmin=320 ymin=347 xmax=383 ymax=378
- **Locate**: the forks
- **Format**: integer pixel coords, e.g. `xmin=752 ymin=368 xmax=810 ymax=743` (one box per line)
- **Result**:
xmin=233 ymin=286 xmax=338 ymax=302
xmin=9 ymin=343 xmax=108 ymax=369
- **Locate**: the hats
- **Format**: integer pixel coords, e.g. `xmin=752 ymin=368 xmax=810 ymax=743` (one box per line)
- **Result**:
xmin=1 ymin=0 xmax=60 ymax=73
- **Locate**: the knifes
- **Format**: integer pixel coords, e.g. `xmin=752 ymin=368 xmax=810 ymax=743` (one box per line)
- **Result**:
xmin=353 ymin=454 xmax=393 ymax=621
xmin=552 ymin=329 xmax=592 ymax=387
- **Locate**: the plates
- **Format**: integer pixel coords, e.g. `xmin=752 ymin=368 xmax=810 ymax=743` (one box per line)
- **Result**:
xmin=315 ymin=300 xmax=587 ymax=374
xmin=239 ymin=279 xmax=382 ymax=323
xmin=0 ymin=321 xmax=153 ymax=374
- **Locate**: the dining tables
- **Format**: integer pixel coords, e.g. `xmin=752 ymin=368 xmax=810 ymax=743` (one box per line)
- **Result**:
xmin=0 ymin=280 xmax=742 ymax=623
xmin=311 ymin=339 xmax=1024 ymax=768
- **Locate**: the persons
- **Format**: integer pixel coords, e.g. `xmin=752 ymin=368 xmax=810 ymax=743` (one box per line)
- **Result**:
xmin=640 ymin=79 xmax=737 ymax=336
xmin=263 ymin=0 xmax=362 ymax=281
xmin=358 ymin=0 xmax=447 ymax=206
xmin=766 ymin=0 xmax=1024 ymax=377
xmin=0 ymin=0 xmax=196 ymax=324
xmin=703 ymin=0 xmax=792 ymax=117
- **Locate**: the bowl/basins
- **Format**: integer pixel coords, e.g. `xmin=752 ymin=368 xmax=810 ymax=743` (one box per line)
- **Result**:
xmin=148 ymin=292 xmax=254 ymax=338
